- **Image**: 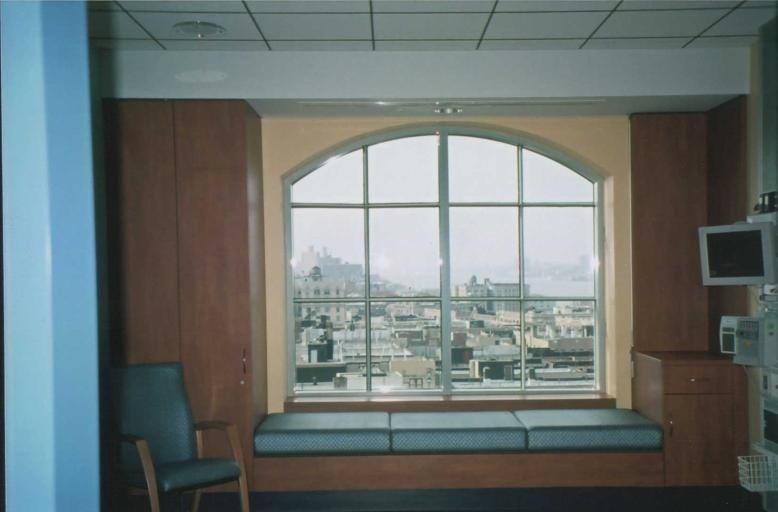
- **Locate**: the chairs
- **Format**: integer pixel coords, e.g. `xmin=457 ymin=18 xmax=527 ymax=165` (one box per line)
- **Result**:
xmin=97 ymin=359 xmax=251 ymax=512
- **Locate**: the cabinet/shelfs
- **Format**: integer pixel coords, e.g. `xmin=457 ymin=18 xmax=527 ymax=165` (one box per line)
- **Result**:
xmin=98 ymin=94 xmax=269 ymax=496
xmin=630 ymin=347 xmax=752 ymax=491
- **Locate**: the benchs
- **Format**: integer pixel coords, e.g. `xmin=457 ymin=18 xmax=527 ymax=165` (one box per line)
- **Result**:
xmin=252 ymin=406 xmax=668 ymax=494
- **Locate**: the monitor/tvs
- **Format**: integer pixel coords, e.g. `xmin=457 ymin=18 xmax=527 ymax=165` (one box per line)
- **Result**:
xmin=698 ymin=221 xmax=778 ymax=286
